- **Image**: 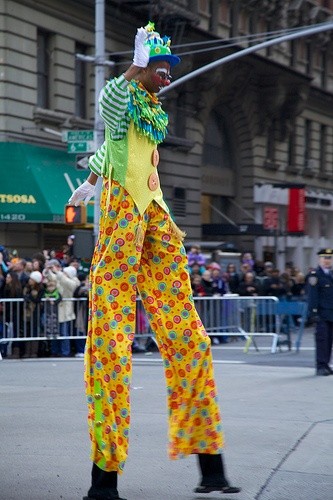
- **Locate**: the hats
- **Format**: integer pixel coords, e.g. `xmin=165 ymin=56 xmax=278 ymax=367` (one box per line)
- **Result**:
xmin=64 ymin=266 xmax=77 ymax=280
xmin=317 ymin=249 xmax=333 ymax=256
xmin=31 ymin=272 xmax=42 ymax=284
xmin=147 ymin=31 xmax=180 ymax=66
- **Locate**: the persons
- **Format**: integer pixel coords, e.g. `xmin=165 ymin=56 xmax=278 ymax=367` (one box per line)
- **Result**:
xmin=68 ymin=22 xmax=243 ymax=500
xmin=0 ymin=235 xmax=314 ymax=360
xmin=307 ymin=248 xmax=333 ymax=376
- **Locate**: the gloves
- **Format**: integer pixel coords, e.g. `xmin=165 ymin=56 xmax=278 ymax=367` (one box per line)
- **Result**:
xmin=68 ymin=181 xmax=95 ymax=207
xmin=132 ymin=27 xmax=151 ymax=68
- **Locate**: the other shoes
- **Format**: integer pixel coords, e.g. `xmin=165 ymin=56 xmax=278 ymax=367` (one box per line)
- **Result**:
xmin=6 ymin=353 xmax=85 ymax=359
xmin=316 ymin=367 xmax=333 ymax=375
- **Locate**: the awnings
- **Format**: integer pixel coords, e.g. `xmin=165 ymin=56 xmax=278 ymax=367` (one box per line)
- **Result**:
xmin=0 ymin=141 xmax=94 ymax=224
